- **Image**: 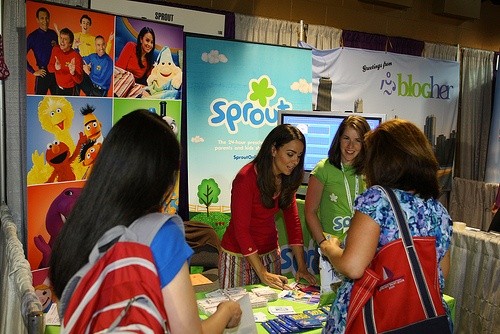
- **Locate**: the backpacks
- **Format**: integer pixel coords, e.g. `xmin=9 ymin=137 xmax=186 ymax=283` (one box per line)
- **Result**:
xmin=59 ymin=212 xmax=186 ymax=334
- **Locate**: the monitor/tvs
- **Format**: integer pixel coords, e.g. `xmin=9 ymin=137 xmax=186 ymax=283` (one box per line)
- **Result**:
xmin=278 ymin=110 xmax=387 ymax=196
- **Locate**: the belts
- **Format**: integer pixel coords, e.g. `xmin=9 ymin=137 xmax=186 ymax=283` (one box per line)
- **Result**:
xmin=39 ymin=66 xmax=48 ymax=68
xmin=94 ymin=83 xmax=105 ymax=92
xmin=59 ymin=86 xmax=66 ymax=91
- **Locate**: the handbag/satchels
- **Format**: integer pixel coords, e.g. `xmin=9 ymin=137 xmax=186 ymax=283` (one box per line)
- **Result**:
xmin=345 ymin=184 xmax=446 ymax=334
xmin=184 ymin=222 xmax=220 ymax=268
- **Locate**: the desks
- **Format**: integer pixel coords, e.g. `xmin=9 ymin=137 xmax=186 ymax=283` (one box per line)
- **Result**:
xmin=42 ymin=274 xmax=456 ymax=334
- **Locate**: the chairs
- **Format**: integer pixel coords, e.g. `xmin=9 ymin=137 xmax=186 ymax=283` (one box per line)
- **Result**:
xmin=183 ymin=219 xmax=222 ymax=292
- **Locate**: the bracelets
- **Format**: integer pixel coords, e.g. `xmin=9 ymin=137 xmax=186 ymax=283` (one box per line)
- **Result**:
xmin=318 ymin=238 xmax=326 ymax=245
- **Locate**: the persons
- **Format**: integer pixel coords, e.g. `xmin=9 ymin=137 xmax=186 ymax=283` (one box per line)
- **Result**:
xmin=216 ymin=123 xmax=317 ymax=290
xmin=115 ymin=26 xmax=155 ymax=83
xmin=304 ymin=115 xmax=372 ymax=252
xmin=47 ymin=109 xmax=242 ymax=334
xmin=317 ymin=119 xmax=456 ymax=332
xmin=27 ymin=6 xmax=113 ymax=97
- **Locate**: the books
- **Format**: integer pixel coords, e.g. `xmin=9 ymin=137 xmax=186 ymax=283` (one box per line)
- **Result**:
xmin=322 ymin=229 xmax=349 ymax=248
